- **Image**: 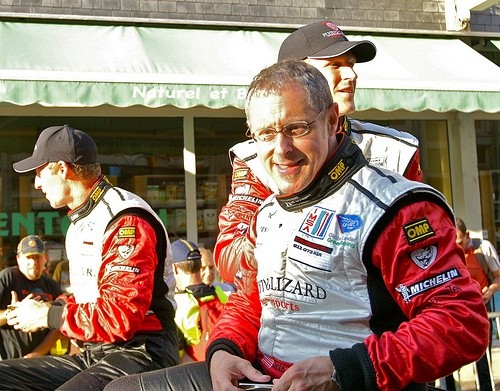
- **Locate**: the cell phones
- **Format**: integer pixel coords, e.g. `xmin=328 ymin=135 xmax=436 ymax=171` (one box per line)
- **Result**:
xmin=239 ymin=383 xmax=275 ymax=391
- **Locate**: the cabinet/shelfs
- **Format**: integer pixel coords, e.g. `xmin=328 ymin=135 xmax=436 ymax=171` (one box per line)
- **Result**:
xmin=480 ymin=170 xmax=500 ymax=256
xmin=19 ymin=174 xmax=227 ymax=262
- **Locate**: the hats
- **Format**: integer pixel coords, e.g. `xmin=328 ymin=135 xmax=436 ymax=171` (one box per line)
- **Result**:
xmin=17 ymin=235 xmax=46 ymax=258
xmin=278 ymin=20 xmax=377 ymax=64
xmin=170 ymin=239 xmax=201 ymax=262
xmin=13 ymin=124 xmax=98 ymax=173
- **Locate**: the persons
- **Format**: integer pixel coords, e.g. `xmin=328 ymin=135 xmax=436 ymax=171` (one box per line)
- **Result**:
xmin=211 ymin=22 xmax=422 ymax=294
xmin=0 ymin=236 xmax=65 ymax=361
xmin=0 ymin=125 xmax=179 ymax=390
xmin=168 ymin=238 xmax=240 ymax=366
xmin=454 ymin=217 xmax=500 ymax=367
xmin=48 ymin=255 xmax=88 ymax=359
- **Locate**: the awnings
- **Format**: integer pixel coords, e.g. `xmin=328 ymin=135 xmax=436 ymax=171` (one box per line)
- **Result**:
xmin=0 ymin=18 xmax=500 ymax=114
xmin=101 ymin=56 xmax=491 ymax=391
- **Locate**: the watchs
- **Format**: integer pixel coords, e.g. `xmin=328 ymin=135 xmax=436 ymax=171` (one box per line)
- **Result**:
xmin=331 ymin=363 xmax=341 ymax=388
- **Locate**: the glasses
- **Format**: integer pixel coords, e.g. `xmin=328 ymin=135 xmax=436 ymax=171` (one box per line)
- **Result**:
xmin=245 ymin=106 xmax=326 ymax=141
